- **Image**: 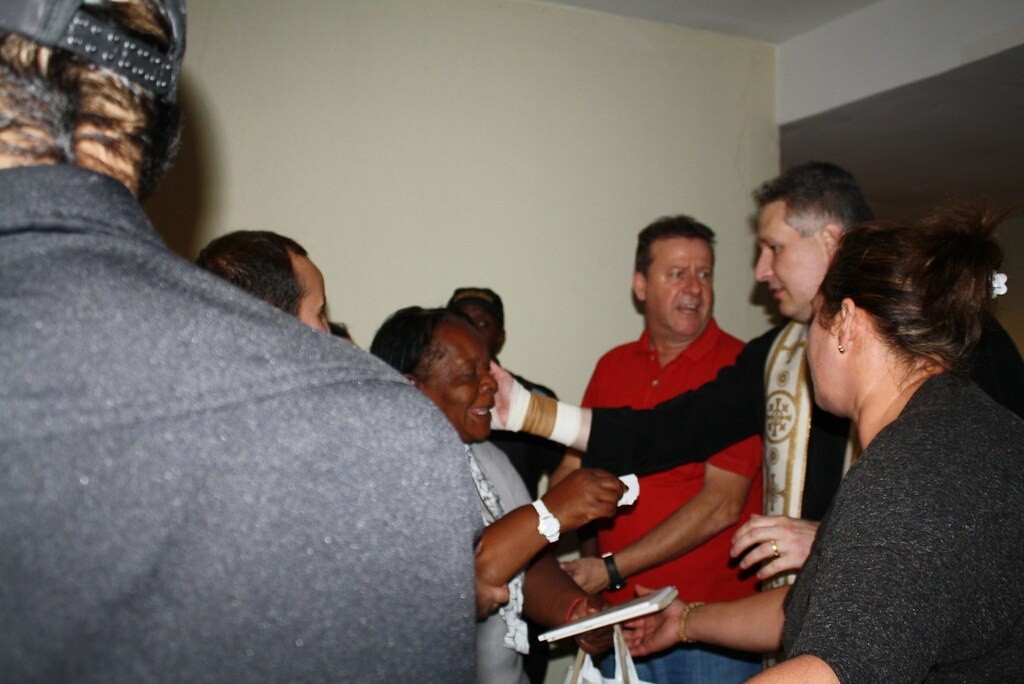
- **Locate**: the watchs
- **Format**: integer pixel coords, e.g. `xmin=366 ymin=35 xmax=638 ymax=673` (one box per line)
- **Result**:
xmin=532 ymin=498 xmax=561 ymax=543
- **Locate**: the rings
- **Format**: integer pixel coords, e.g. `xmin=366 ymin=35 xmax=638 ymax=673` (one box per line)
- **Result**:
xmin=770 ymin=539 xmax=780 ymax=556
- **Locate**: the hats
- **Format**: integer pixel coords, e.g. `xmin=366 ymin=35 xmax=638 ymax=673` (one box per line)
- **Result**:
xmin=0 ymin=0 xmax=186 ymax=102
xmin=447 ymin=286 xmax=505 ymax=328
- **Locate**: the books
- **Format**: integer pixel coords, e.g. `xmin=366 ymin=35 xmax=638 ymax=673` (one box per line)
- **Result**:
xmin=538 ymin=587 xmax=678 ymax=644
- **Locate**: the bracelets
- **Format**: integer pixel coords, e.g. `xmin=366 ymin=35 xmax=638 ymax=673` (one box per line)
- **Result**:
xmin=564 ymin=596 xmax=584 ymax=625
xmin=679 ymin=601 xmax=705 ymax=643
xmin=602 ymin=552 xmax=627 ymax=590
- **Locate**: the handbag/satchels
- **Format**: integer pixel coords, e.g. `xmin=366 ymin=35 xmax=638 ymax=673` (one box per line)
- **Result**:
xmin=563 ymin=625 xmax=653 ymax=684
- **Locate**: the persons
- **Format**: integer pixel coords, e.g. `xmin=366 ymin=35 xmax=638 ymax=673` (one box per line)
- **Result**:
xmin=368 ymin=306 xmax=628 ymax=684
xmin=490 ymin=159 xmax=1023 ymax=669
xmin=621 ymin=197 xmax=1024 ymax=684
xmin=198 ymin=229 xmax=509 ymax=626
xmin=446 ymin=284 xmax=568 ymax=504
xmin=0 ymin=0 xmax=481 ymax=684
xmin=548 ymin=214 xmax=766 ymax=684
